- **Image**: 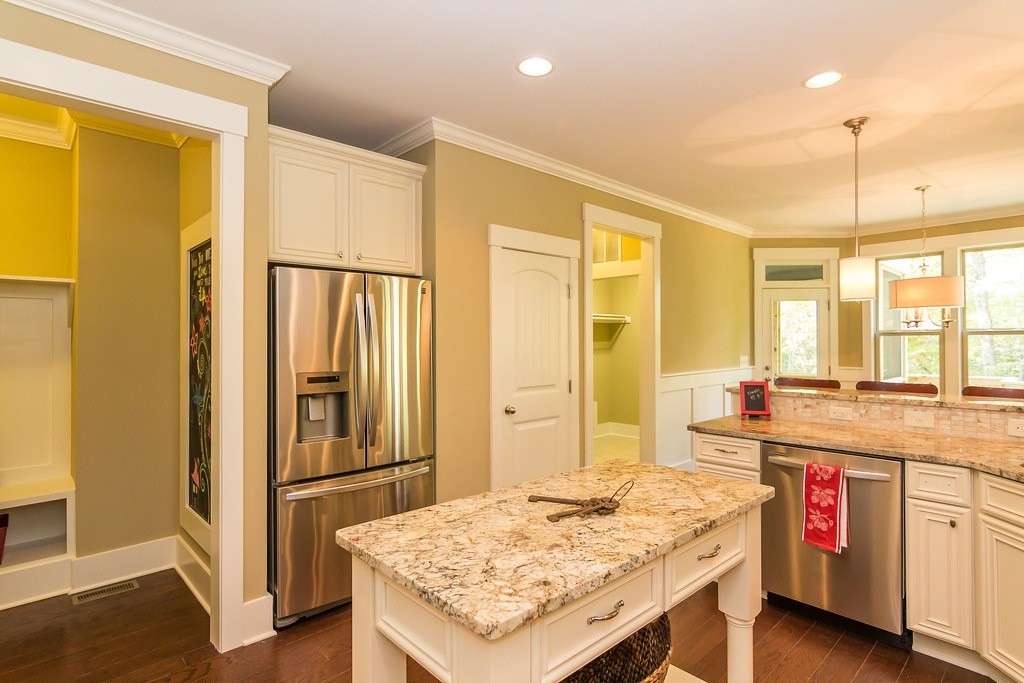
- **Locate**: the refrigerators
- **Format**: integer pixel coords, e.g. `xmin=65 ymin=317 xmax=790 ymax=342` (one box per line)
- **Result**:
xmin=268 ymin=264 xmax=434 ymax=619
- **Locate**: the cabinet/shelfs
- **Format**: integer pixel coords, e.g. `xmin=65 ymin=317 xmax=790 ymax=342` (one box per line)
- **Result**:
xmin=266 ymin=124 xmax=429 ymax=278
xmin=695 ymin=432 xmax=1024 ymax=683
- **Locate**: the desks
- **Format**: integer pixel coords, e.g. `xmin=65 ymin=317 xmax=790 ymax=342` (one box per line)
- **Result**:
xmin=336 ymin=460 xmax=775 ymax=683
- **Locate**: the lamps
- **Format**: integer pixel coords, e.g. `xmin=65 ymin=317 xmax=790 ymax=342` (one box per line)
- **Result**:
xmin=888 ymin=185 xmax=964 ymax=328
xmin=839 ymin=116 xmax=875 ymax=301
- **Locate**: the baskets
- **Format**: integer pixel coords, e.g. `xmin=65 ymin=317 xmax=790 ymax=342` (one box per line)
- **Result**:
xmin=560 ymin=612 xmax=673 ymax=683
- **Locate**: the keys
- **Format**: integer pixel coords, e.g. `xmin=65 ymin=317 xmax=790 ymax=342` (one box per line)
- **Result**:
xmin=528 ymin=496 xmax=621 ymax=522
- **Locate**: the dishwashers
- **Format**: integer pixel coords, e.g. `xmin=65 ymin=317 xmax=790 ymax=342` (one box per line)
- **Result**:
xmin=758 ymin=442 xmax=905 ymax=638
